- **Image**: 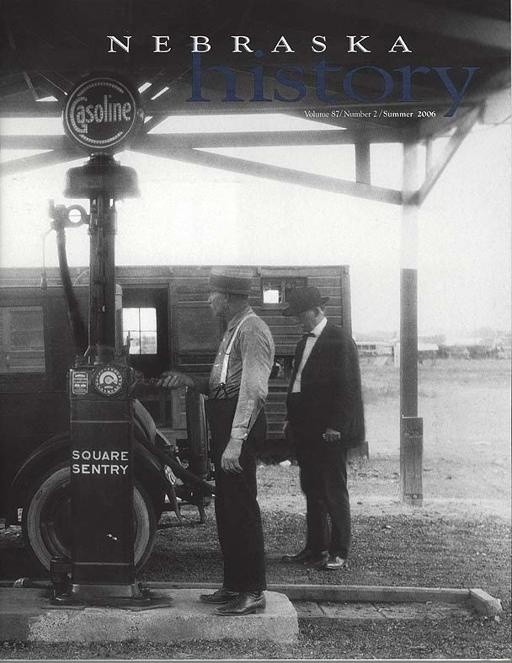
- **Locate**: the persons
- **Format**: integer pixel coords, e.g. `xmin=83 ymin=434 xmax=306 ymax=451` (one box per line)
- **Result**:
xmin=154 ymin=264 xmax=275 ymax=616
xmin=281 ymin=283 xmax=365 ymax=569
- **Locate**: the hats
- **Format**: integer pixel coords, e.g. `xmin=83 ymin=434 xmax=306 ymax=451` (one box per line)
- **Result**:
xmin=282 ymin=287 xmax=330 ymax=317
xmin=208 ymin=270 xmax=252 ymax=296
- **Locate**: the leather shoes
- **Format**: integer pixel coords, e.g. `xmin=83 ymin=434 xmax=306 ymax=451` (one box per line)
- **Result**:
xmin=200 ymin=589 xmax=238 ymax=603
xmin=214 ymin=592 xmax=266 ymax=615
xmin=282 ymin=546 xmax=329 ymax=564
xmin=325 ymin=553 xmax=349 ymax=569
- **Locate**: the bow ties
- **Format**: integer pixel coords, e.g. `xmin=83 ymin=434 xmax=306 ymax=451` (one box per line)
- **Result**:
xmin=302 ymin=333 xmax=315 ymax=338
